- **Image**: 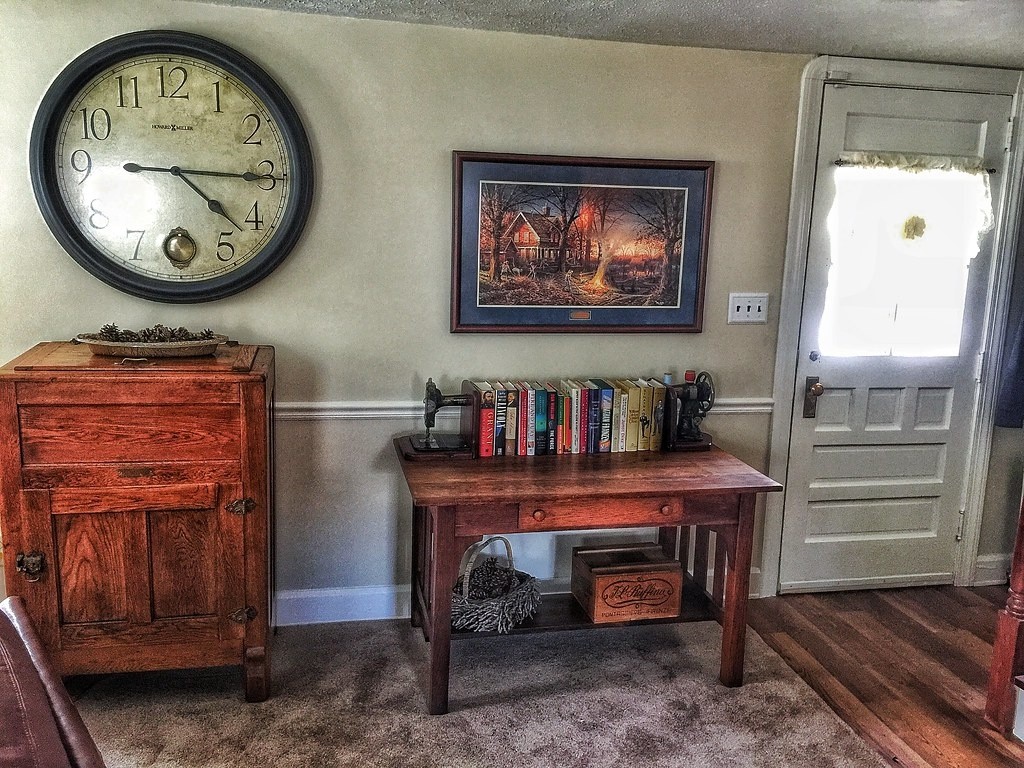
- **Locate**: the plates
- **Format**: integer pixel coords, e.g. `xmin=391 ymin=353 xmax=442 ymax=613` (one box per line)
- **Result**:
xmin=74 ymin=333 xmax=230 ymax=358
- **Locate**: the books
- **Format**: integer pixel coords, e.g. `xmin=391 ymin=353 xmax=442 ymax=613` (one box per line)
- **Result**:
xmin=474 ymin=378 xmax=667 ymax=457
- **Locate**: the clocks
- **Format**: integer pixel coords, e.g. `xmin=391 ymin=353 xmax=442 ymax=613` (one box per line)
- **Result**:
xmin=29 ymin=28 xmax=315 ymax=304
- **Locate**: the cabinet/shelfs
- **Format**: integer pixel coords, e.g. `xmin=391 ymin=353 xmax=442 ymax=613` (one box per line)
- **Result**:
xmin=1 ymin=341 xmax=274 ymax=707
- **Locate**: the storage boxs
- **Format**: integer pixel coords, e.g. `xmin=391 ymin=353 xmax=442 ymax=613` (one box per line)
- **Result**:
xmin=571 ymin=541 xmax=690 ymax=625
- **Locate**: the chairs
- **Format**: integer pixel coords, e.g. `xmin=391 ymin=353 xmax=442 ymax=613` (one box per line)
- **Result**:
xmin=0 ymin=596 xmax=108 ymax=768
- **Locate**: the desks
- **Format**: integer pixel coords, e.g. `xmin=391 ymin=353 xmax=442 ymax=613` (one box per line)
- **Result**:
xmin=389 ymin=436 xmax=787 ymax=697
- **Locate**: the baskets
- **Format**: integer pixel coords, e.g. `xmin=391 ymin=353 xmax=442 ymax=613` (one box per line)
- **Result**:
xmin=451 ymin=536 xmax=542 ymax=633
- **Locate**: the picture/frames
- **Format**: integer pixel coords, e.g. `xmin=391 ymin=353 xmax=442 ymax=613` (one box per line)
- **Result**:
xmin=452 ymin=152 xmax=717 ymax=333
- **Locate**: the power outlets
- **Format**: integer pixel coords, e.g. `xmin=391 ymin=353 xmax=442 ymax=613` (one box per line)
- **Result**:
xmin=727 ymin=292 xmax=769 ymax=325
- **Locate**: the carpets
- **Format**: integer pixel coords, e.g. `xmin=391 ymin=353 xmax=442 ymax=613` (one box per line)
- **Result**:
xmin=32 ymin=613 xmax=899 ymax=768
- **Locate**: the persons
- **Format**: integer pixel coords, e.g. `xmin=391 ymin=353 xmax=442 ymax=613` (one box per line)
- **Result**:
xmin=528 ymin=441 xmax=534 ymax=448
xmin=481 ymin=391 xmax=494 ymax=408
xmin=508 ymin=392 xmax=516 ymax=406
xmin=652 ymin=400 xmax=664 ymax=436
xmin=640 ymin=412 xmax=649 ymax=437
